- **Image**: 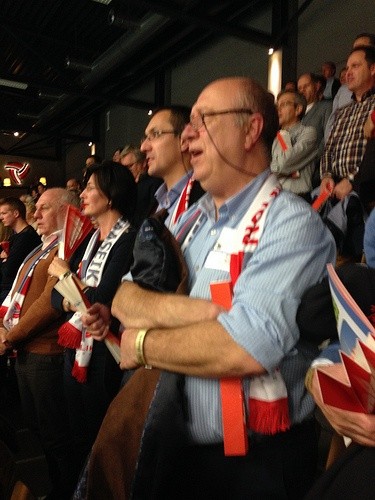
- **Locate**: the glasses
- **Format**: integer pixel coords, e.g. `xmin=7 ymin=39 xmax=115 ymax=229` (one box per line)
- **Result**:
xmin=190 ymin=108 xmax=252 ymax=129
xmin=140 ymin=129 xmax=179 ymax=142
